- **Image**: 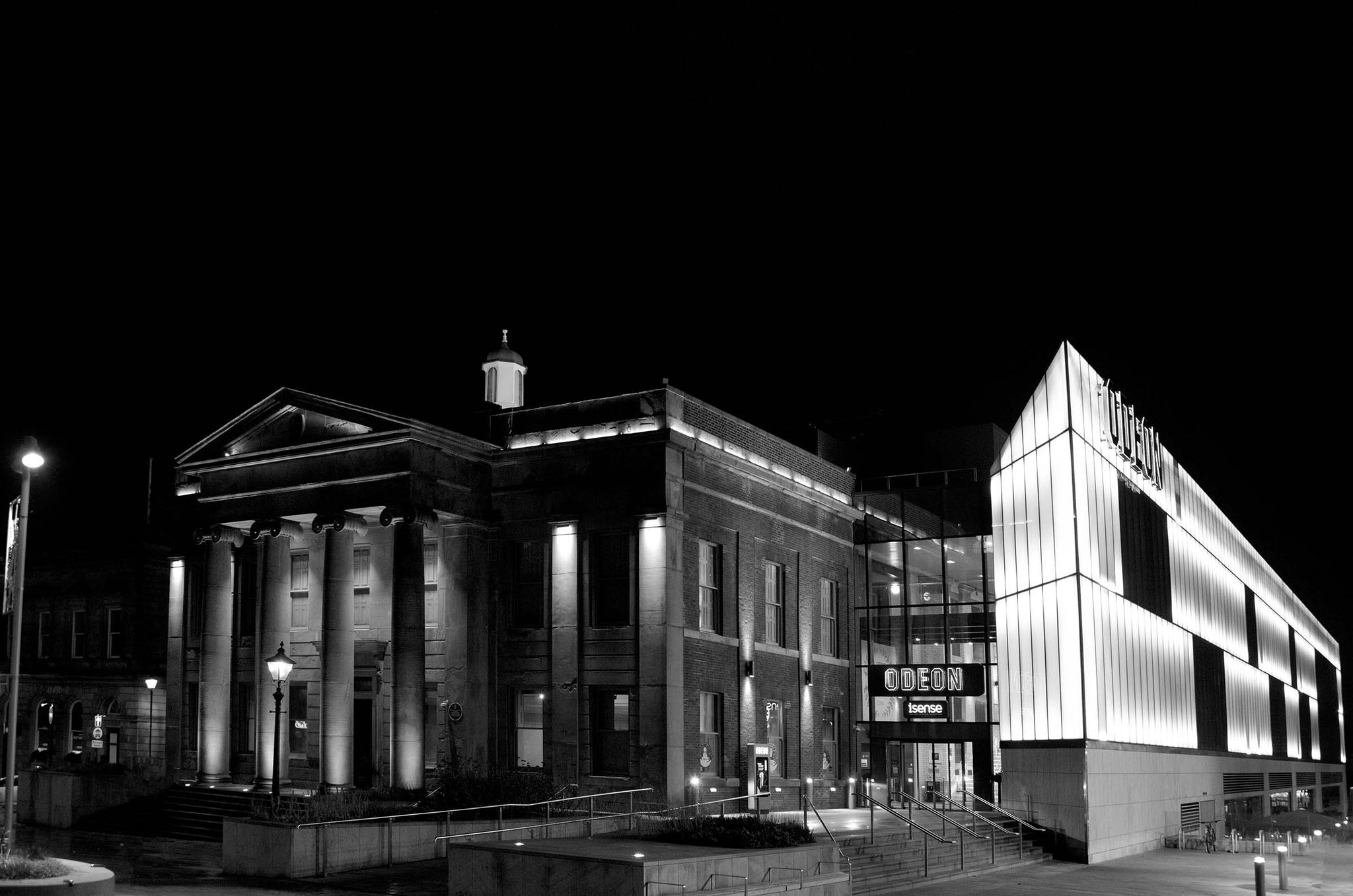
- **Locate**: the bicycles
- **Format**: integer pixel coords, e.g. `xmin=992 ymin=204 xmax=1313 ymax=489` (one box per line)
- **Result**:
xmin=1200 ymin=820 xmax=1222 ymax=853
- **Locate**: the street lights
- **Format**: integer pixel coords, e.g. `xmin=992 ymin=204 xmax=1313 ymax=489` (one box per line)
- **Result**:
xmin=1 ymin=434 xmax=46 ymax=859
xmin=145 ymin=678 xmax=158 ymax=765
xmin=262 ymin=639 xmax=296 ymax=810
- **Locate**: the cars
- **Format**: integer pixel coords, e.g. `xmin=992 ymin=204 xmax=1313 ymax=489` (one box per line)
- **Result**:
xmin=0 ymin=775 xmax=19 ymax=807
